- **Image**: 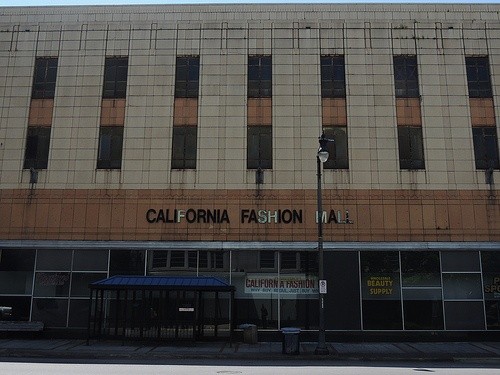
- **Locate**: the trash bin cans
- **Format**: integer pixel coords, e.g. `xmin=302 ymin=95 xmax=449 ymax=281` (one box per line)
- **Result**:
xmin=281 ymin=327 xmax=301 ymax=355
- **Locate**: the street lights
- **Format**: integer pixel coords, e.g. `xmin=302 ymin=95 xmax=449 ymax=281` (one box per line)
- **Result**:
xmin=314 ymin=135 xmax=335 ymax=354
